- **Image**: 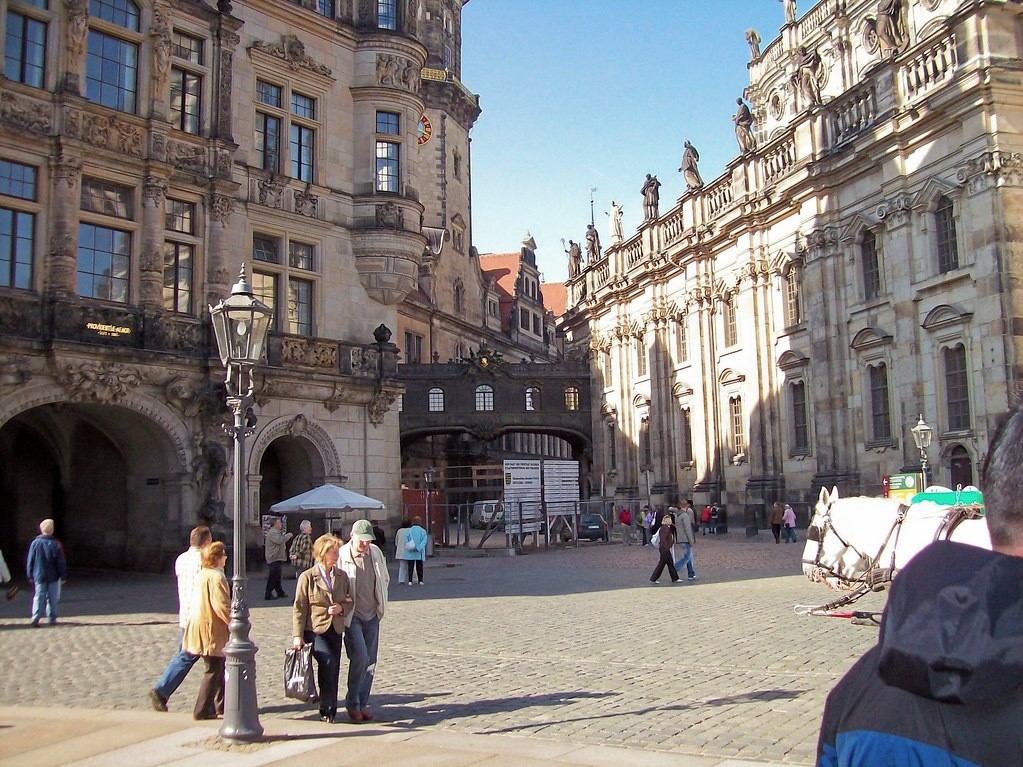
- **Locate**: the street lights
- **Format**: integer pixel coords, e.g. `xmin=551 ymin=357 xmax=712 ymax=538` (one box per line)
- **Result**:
xmin=911 ymin=412 xmax=934 ymax=493
xmin=423 ymin=466 xmax=437 ymax=534
xmin=209 ymin=262 xmax=275 ymax=746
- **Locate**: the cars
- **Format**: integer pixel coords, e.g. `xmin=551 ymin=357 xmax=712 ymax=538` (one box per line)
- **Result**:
xmin=560 ymin=514 xmax=609 ymax=542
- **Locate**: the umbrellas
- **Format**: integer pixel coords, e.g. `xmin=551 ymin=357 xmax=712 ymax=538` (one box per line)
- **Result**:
xmin=268 ymin=482 xmax=388 ymax=532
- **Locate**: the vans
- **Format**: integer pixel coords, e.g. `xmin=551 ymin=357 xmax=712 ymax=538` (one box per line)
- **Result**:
xmin=470 ymin=500 xmax=505 ymax=529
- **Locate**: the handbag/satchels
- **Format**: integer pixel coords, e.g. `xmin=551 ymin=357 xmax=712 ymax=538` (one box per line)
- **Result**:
xmin=285 ymin=642 xmax=320 ymax=705
xmin=651 ymin=527 xmax=662 ymax=548
xmin=6 ymin=584 xmax=20 ymax=600
xmin=405 ymin=529 xmax=416 ymax=551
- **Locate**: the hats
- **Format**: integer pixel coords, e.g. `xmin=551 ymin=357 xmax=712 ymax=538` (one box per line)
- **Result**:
xmin=353 ymin=519 xmax=377 ymax=541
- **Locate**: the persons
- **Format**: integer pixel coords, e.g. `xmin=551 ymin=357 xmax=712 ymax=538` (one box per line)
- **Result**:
xmin=333 ymin=520 xmax=390 ymax=723
xmin=730 ymin=97 xmax=757 ymax=153
xmin=376 ymin=52 xmax=417 ymax=94
xmin=678 ymin=138 xmax=704 ymax=192
xmin=875 ymin=0 xmax=905 ymax=52
xmin=815 ymin=405 xmax=1023 ymax=767
xmin=264 ymin=517 xmax=428 ymax=601
xmin=649 ymin=514 xmax=684 ymax=585
xmin=564 ymin=239 xmax=582 ymax=279
xmin=291 ymin=533 xmax=354 ymax=726
xmin=147 ymin=525 xmax=232 ymax=721
xmin=640 ymin=173 xmax=662 ymax=221
xmin=778 ymin=0 xmax=797 ymax=25
xmin=604 ymin=200 xmax=625 ymax=244
xmin=768 ymin=502 xmax=798 ymax=544
xmin=744 ymin=28 xmax=762 ymax=60
xmin=668 ymin=500 xmax=701 ymax=582
xmin=584 ymin=225 xmax=602 ymax=265
xmin=148 ymin=8 xmax=172 ymax=83
xmin=0 ymin=95 xmax=206 ymax=172
xmin=0 ymin=518 xmax=69 ymax=628
xmin=619 ymin=502 xmax=720 ymax=547
xmin=789 ymin=41 xmax=824 ymax=109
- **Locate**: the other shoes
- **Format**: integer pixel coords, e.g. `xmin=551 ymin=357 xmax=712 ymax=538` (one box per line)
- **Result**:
xmin=265 ymin=595 xmax=278 ymax=600
xmin=277 ymin=593 xmax=288 ymax=597
xmin=348 ymin=709 xmax=373 ymax=722
xmin=689 ymin=576 xmax=699 ymax=580
xmin=150 ymin=689 xmax=168 ymax=712
xmin=408 ymin=582 xmax=412 ymax=585
xmin=654 ymin=580 xmax=660 ymax=584
xmin=675 ymin=578 xmax=683 ymax=582
xmin=321 ymin=714 xmax=335 ymax=723
xmin=419 ymin=582 xmax=425 ymax=585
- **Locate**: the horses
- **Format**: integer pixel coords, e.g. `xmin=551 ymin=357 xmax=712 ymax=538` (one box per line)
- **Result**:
xmin=802 ymin=485 xmax=992 ymax=587
